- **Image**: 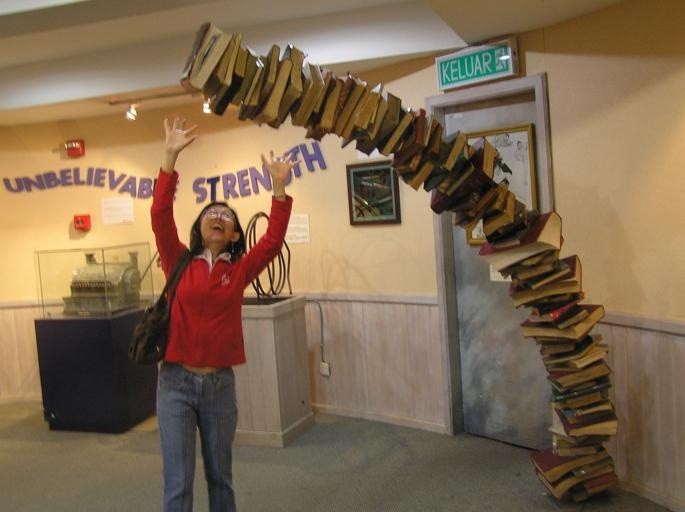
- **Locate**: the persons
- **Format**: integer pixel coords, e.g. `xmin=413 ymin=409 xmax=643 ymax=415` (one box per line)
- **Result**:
xmin=150 ymin=115 xmax=297 ymax=511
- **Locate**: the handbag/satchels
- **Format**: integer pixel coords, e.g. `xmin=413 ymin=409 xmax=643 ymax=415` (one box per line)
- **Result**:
xmin=124 ymin=246 xmax=194 ymax=365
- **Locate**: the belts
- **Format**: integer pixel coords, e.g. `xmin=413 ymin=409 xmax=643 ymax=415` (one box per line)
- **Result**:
xmin=179 ymin=363 xmax=225 ymax=376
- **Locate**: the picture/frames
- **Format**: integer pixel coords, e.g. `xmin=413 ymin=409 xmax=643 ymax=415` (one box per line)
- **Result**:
xmin=463 ymin=122 xmax=537 ymax=246
xmin=345 ymin=159 xmax=402 ymax=227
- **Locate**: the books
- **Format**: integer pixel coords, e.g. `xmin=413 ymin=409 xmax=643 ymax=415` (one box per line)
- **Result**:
xmin=179 ymin=21 xmax=380 ymax=156
xmin=509 ymin=253 xmax=621 ymax=506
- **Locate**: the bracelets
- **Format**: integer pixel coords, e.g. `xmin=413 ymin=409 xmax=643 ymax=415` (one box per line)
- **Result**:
xmin=273 ymin=193 xmax=287 ymax=199
